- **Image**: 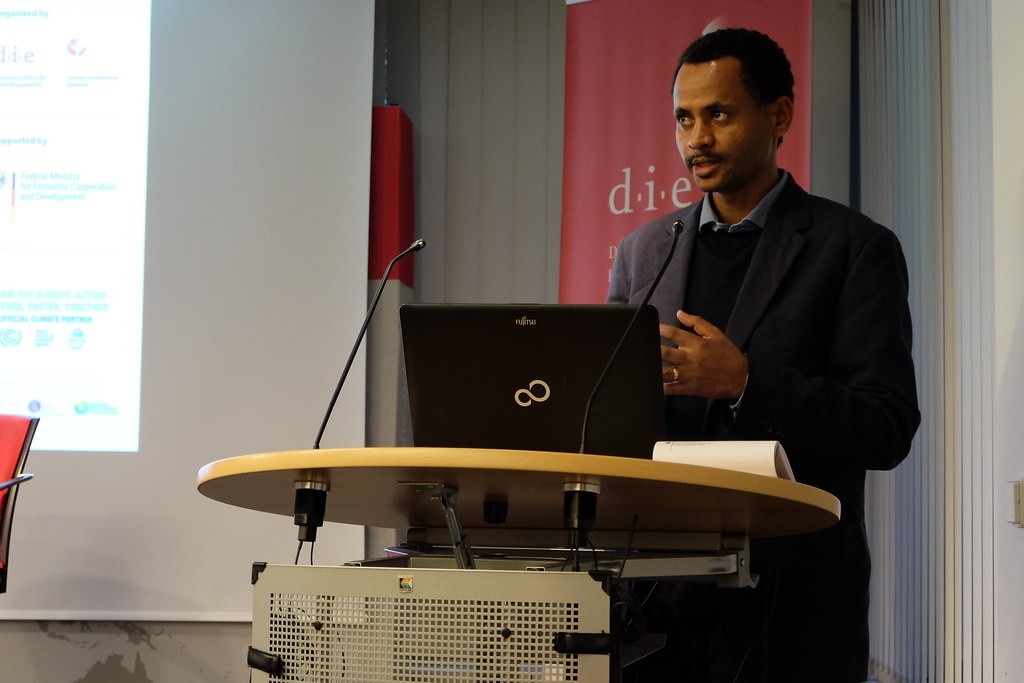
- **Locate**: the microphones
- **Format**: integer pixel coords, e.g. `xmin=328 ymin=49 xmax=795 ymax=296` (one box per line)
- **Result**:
xmin=580 ymin=221 xmax=685 ymax=454
xmin=312 ymin=238 xmax=427 ymax=449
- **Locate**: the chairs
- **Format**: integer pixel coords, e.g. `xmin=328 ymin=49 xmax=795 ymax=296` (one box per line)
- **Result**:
xmin=0 ymin=412 xmax=41 ymax=595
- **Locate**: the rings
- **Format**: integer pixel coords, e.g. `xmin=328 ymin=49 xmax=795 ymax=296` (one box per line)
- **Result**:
xmin=673 ymin=365 xmax=677 ymax=381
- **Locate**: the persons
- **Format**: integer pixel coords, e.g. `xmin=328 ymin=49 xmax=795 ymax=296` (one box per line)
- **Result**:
xmin=602 ymin=27 xmax=922 ymax=683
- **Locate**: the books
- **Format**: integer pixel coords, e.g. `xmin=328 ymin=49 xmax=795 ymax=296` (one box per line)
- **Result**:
xmin=653 ymin=439 xmax=795 ymax=482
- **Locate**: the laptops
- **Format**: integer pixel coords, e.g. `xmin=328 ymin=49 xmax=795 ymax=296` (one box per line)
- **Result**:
xmin=399 ymin=301 xmax=666 ymax=461
